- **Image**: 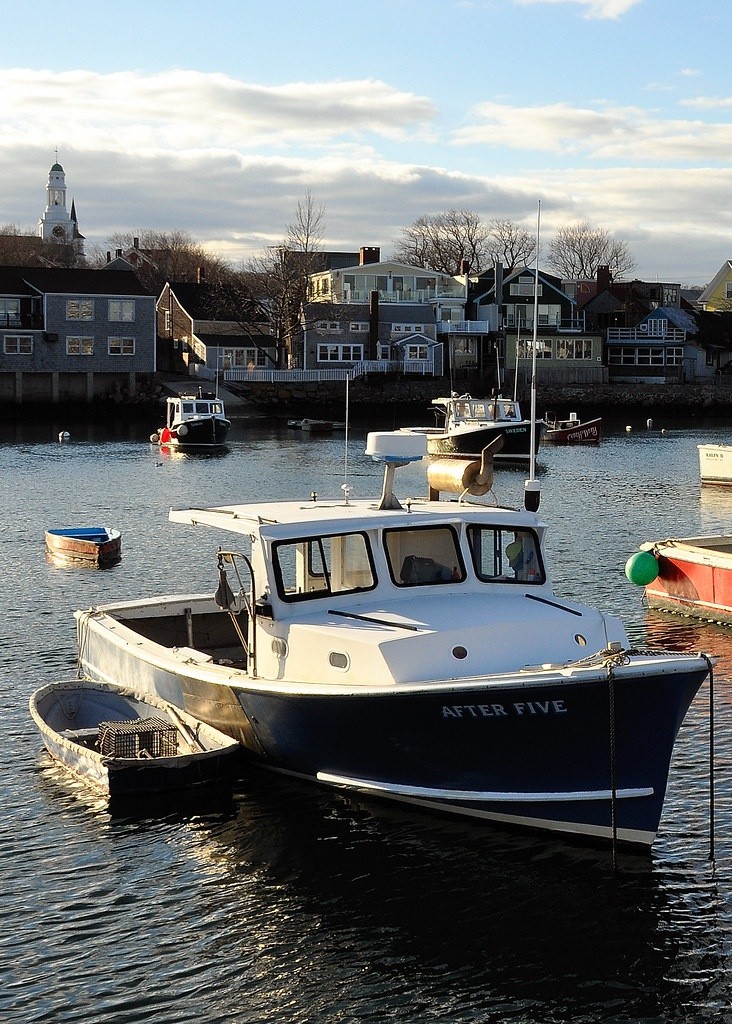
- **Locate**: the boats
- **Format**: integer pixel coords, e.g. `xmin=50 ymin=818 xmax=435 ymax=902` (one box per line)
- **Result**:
xmin=639 ymin=535 xmax=732 ymax=624
xmin=157 ymin=387 xmax=231 ymax=446
xmin=541 ymin=412 xmax=603 ymax=445
xmin=29 ymin=679 xmax=237 ymax=802
xmin=287 ymin=419 xmax=351 ymax=433
xmin=45 ymin=528 xmax=121 ymax=560
xmin=72 ymin=430 xmax=718 ymax=847
xmin=697 ymin=443 xmax=732 ymax=485
xmin=400 ymin=388 xmax=545 ymax=460
